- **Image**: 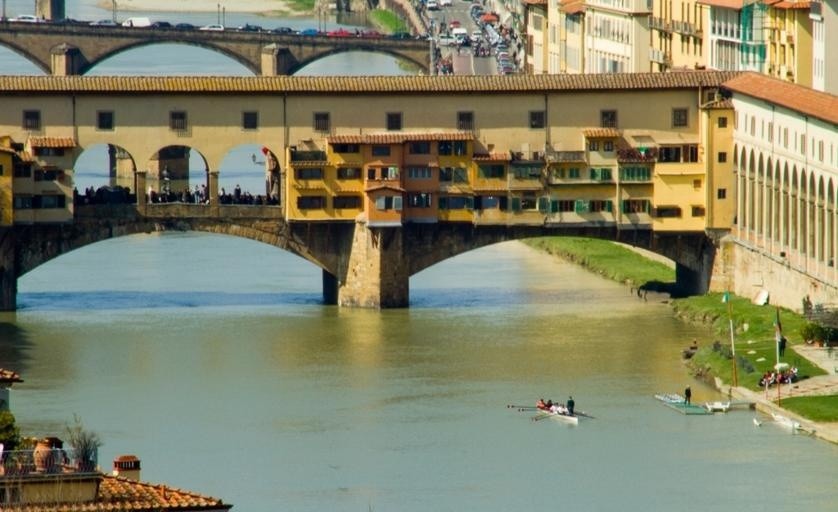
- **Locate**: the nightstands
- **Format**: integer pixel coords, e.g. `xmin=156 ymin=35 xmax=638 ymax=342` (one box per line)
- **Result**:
xmin=507 ymin=404 xmax=557 ymax=421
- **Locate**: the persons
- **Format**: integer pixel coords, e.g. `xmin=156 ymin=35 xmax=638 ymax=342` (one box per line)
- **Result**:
xmin=435 ymin=56 xmax=454 ymax=77
xmin=684 ymin=385 xmax=691 ymax=407
xmin=498 ymin=24 xmax=524 ymax=65
xmin=455 ymin=34 xmax=491 ymax=56
xmin=778 ymin=334 xmax=786 ymax=357
xmin=759 ymin=367 xmax=798 ymax=388
xmin=536 ymin=396 xmax=575 ymax=417
xmin=72 ymin=184 xmax=281 ymax=207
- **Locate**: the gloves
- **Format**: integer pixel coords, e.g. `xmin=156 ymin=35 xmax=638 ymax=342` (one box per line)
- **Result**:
xmin=655 ymin=394 xmax=713 ymax=415
xmin=536 ymin=402 xmax=579 ymax=424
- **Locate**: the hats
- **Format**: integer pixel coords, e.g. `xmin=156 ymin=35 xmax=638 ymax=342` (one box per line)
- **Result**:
xmin=296 ymin=28 xmax=317 ymax=35
xmin=327 ymin=28 xmax=354 ymax=37
xmin=494 ymin=40 xmax=514 ymax=75
xmin=360 ymin=30 xmax=431 ymax=41
xmin=89 ymin=18 xmax=115 ymax=26
xmin=122 ymin=18 xmax=223 ymax=32
xmin=439 ymin=22 xmax=489 ymax=56
xmin=268 ymin=27 xmax=291 ymax=34
xmin=470 ymin=5 xmax=485 ymax=25
xmin=9 ymin=15 xmax=43 ymax=23
xmin=47 ymin=17 xmax=85 ymax=25
xmin=237 ymin=25 xmax=261 ymax=31
xmin=427 ymin=0 xmax=452 ymax=10
